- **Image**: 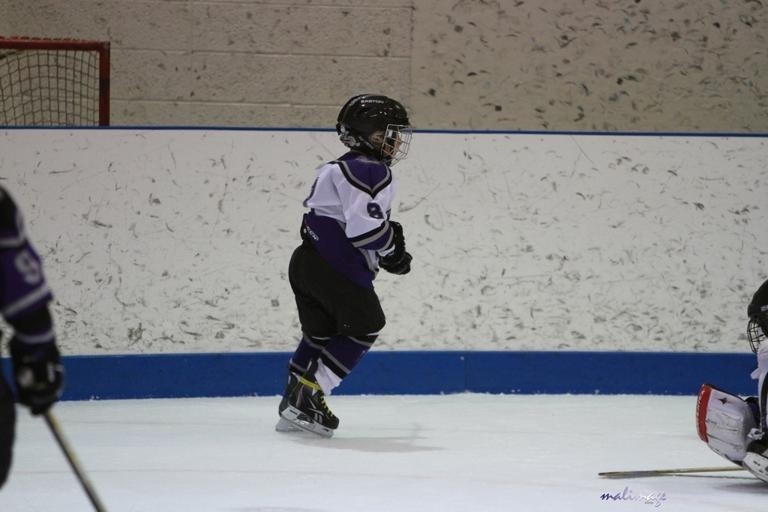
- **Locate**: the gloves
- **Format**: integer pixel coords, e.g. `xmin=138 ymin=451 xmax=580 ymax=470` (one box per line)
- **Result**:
xmin=6 ymin=333 xmax=67 ymax=418
xmin=375 ymin=246 xmax=412 ymax=275
xmin=389 ymin=220 xmax=406 ymax=246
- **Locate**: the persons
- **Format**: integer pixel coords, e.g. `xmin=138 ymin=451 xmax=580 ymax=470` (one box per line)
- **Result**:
xmin=282 ymin=91 xmax=415 ymax=430
xmin=693 ymin=278 xmax=767 ymax=483
xmin=0 ymin=184 xmax=65 ymax=489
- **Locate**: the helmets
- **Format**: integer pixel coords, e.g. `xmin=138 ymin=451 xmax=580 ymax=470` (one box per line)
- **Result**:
xmin=746 ymin=278 xmax=768 ymax=336
xmin=333 ymin=94 xmax=409 ymax=136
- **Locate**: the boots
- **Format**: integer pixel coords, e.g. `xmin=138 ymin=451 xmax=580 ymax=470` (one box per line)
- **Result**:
xmin=277 ymin=358 xmax=306 ymax=415
xmin=287 ymin=358 xmax=340 ymax=429
